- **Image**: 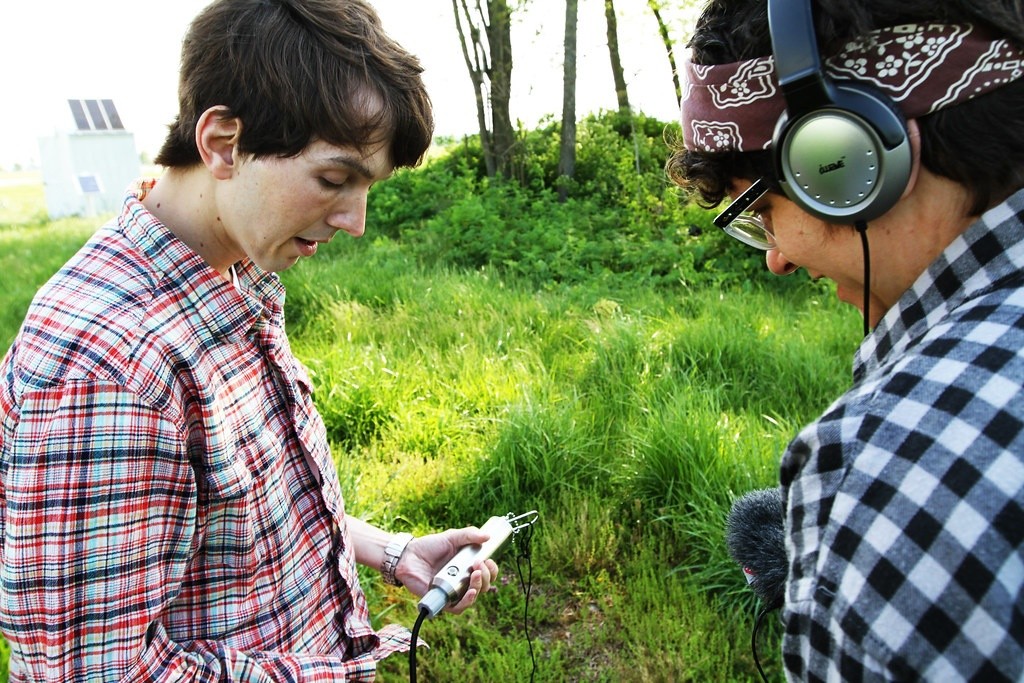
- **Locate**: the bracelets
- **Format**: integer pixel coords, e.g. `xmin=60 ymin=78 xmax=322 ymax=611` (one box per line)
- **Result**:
xmin=381 ymin=532 xmax=417 ymax=589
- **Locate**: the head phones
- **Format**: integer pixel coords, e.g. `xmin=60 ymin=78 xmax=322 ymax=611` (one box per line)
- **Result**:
xmin=763 ymin=0 xmax=913 ymax=226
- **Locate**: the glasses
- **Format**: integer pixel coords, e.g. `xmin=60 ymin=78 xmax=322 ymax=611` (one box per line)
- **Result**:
xmin=712 ymin=177 xmax=777 ymax=250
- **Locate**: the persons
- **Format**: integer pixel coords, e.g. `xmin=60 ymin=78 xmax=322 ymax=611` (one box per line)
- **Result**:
xmin=0 ymin=1 xmax=501 ymax=683
xmin=662 ymin=0 xmax=1024 ymax=683
